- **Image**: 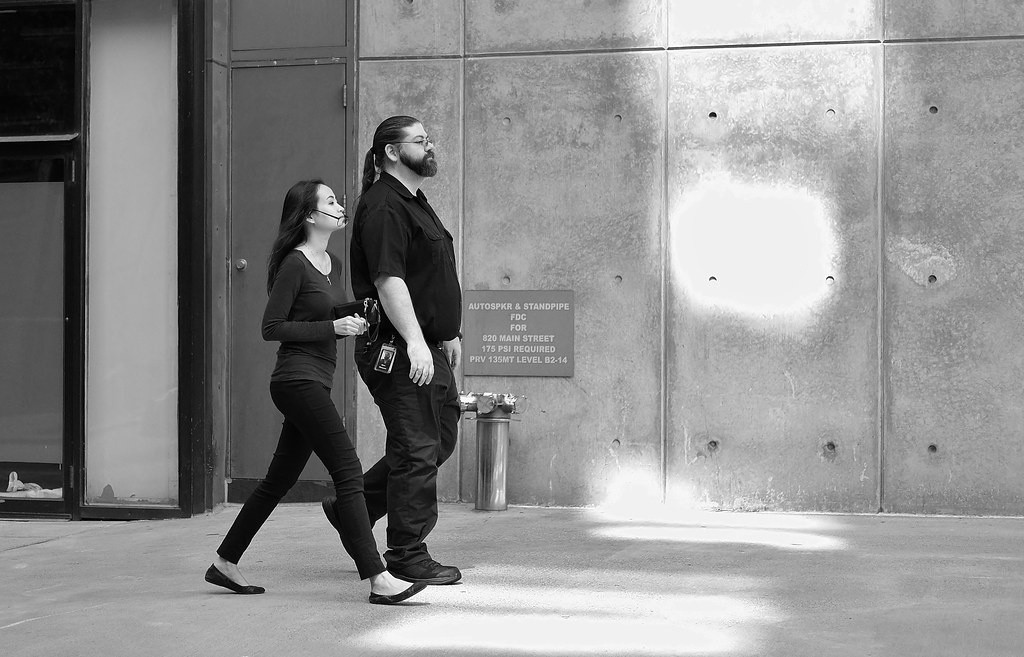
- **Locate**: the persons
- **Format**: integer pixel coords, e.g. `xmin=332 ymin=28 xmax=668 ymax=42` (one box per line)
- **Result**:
xmin=320 ymin=116 xmax=465 ymax=587
xmin=377 ymin=352 xmax=390 ymax=370
xmin=202 ymin=180 xmax=428 ymax=603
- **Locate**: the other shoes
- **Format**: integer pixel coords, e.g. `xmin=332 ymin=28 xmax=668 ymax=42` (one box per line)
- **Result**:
xmin=204 ymin=563 xmax=265 ymax=594
xmin=368 ymin=578 xmax=427 ymax=604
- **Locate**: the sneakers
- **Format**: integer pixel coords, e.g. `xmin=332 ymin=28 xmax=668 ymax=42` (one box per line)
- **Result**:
xmin=322 ymin=495 xmax=342 ymax=535
xmin=386 ymin=554 xmax=461 ymax=584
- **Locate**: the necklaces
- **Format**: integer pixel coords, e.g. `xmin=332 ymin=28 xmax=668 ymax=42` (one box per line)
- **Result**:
xmin=301 ymin=241 xmax=332 ymax=285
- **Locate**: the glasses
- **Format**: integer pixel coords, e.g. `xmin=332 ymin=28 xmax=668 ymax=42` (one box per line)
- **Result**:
xmin=310 ymin=209 xmax=349 ymax=228
xmin=384 ymin=135 xmax=433 ymax=147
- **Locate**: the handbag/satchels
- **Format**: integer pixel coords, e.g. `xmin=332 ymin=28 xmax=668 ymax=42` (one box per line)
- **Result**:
xmin=333 ymin=297 xmax=381 ymax=343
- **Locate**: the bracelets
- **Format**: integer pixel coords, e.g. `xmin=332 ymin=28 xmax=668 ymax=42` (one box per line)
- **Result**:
xmin=456 ymin=331 xmax=464 ymax=341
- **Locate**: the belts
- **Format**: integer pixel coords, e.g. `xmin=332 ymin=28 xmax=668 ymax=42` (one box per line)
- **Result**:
xmin=379 ymin=330 xmax=444 ymax=350
